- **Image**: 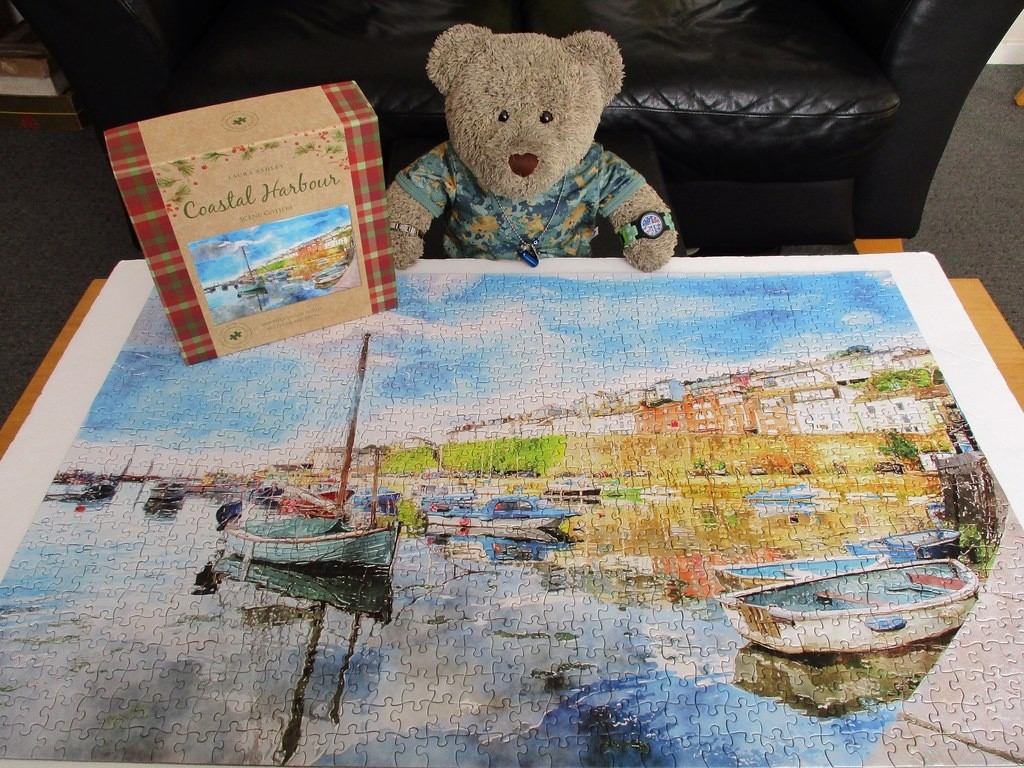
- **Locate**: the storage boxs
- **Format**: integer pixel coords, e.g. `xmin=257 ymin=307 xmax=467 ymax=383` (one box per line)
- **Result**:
xmin=104 ymin=80 xmax=397 ymax=366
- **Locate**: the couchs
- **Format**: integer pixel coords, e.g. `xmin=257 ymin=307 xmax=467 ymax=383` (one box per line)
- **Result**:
xmin=14 ymin=0 xmax=1023 ymax=254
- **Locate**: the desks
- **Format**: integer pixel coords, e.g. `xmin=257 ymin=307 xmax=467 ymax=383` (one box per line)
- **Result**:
xmin=0 ymin=278 xmax=1024 ymax=458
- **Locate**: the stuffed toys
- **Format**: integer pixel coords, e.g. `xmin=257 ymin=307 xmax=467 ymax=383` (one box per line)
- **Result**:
xmin=384 ymin=24 xmax=679 ymax=273
